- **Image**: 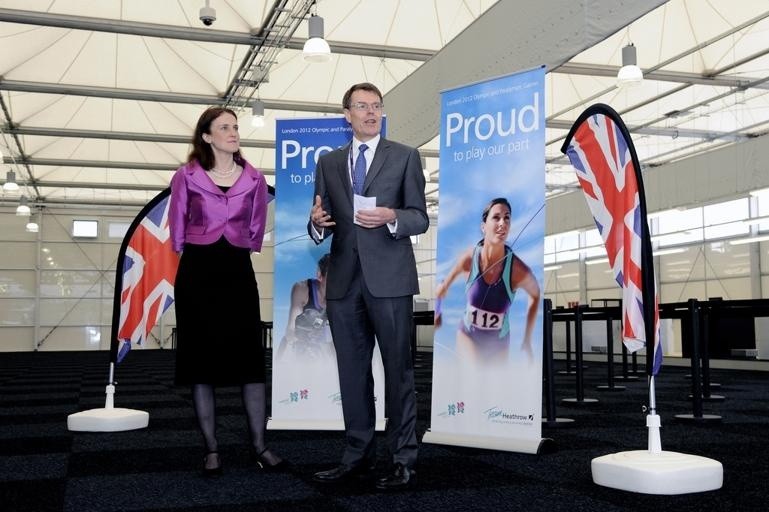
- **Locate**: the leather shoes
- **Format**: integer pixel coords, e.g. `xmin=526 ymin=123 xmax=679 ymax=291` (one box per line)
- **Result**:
xmin=311 ymin=462 xmax=376 ymax=484
xmin=376 ymin=465 xmax=418 ymax=490
xmin=202 ymin=450 xmax=224 ymax=479
xmin=254 ymin=447 xmax=292 ymax=473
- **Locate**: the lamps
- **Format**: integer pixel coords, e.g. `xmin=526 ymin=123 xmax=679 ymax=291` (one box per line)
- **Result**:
xmin=16 ymin=194 xmax=31 ymax=214
xmin=617 ymin=42 xmax=642 ymax=80
xmin=2 ymin=164 xmax=19 ymax=191
xmin=240 ymin=82 xmax=271 ymax=130
xmin=300 ymin=11 xmax=334 ymax=60
xmin=26 ymin=214 xmax=39 ymax=234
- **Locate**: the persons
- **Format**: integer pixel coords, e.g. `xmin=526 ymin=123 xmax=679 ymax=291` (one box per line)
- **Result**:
xmin=279 ymin=252 xmax=335 ymax=368
xmin=310 ymin=84 xmax=430 ymax=490
xmin=168 ymin=108 xmax=285 ymax=478
xmin=434 ymin=198 xmax=540 ymax=369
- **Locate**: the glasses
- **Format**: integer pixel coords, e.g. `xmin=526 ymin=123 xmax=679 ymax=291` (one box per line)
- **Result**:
xmin=349 ymin=103 xmax=384 ymax=109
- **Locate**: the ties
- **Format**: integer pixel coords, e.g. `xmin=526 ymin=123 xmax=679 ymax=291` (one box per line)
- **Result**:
xmin=353 ymin=144 xmax=369 ymax=194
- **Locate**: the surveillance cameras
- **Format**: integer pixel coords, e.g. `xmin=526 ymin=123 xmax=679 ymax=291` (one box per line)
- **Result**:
xmin=199 ymin=7 xmax=216 ymax=25
